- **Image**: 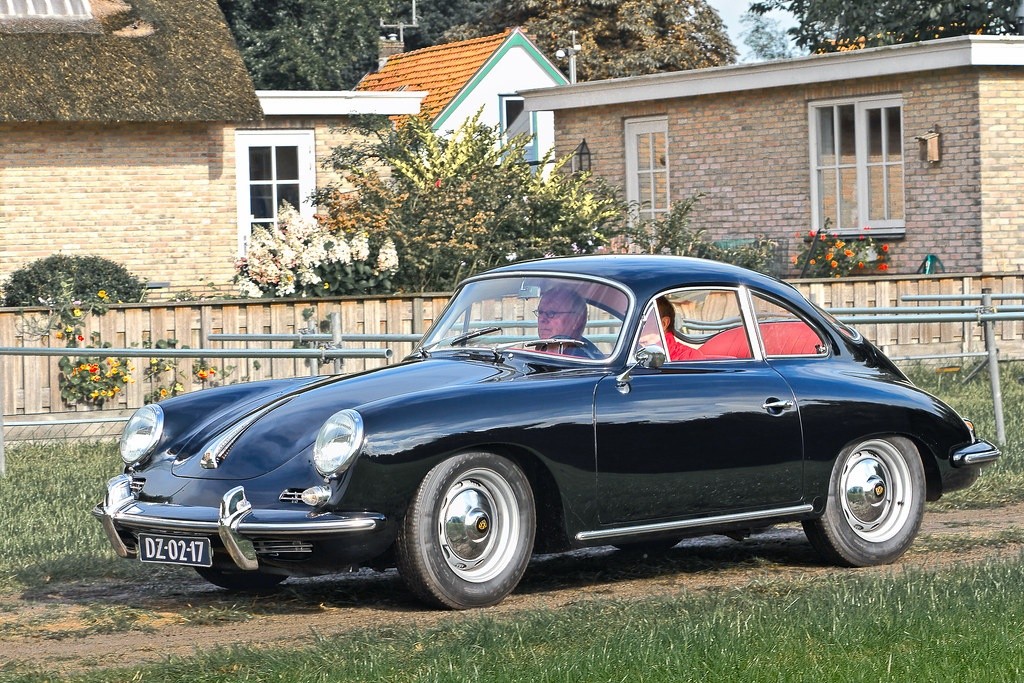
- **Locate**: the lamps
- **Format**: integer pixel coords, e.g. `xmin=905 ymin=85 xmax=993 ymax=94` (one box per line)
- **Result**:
xmin=569 ymin=138 xmax=592 ymax=176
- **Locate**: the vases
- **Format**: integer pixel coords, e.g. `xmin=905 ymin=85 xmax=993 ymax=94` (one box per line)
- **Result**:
xmin=858 ymin=246 xmax=877 ymax=262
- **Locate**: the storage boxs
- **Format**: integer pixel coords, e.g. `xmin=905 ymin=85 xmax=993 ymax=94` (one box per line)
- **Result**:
xmin=915 ymin=132 xmax=940 ymax=163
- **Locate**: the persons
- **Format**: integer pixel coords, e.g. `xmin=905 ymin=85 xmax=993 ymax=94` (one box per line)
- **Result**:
xmin=634 ymin=295 xmax=703 ymax=361
xmin=531 ymin=284 xmax=604 ymax=360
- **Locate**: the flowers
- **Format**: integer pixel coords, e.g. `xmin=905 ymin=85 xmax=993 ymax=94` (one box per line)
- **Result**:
xmin=845 ymin=235 xmax=891 ymax=270
xmin=792 ymin=230 xmax=846 ymax=278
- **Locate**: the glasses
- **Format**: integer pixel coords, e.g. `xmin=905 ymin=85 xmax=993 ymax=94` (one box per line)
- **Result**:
xmin=532 ymin=309 xmax=577 ymax=318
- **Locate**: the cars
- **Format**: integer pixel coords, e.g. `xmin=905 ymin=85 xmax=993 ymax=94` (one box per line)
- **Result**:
xmin=89 ymin=252 xmax=1001 ymax=612
